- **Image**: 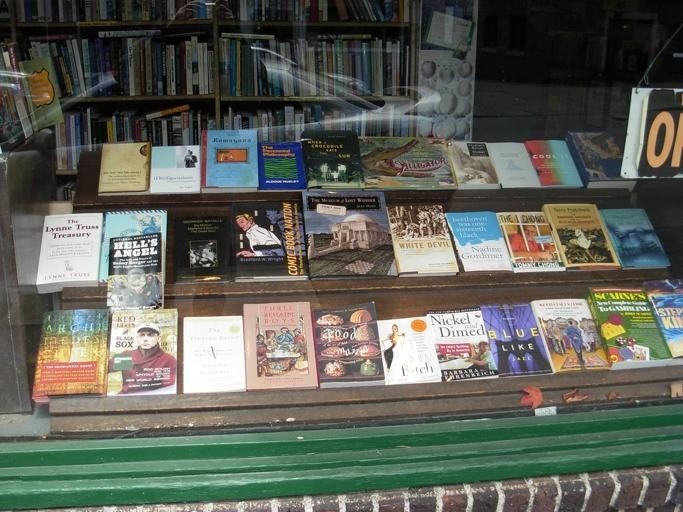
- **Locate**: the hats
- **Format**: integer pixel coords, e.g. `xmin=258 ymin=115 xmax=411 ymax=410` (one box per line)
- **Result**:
xmin=137 ymin=322 xmax=160 ymax=335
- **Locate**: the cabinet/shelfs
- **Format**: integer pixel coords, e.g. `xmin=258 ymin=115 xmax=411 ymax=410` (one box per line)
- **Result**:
xmin=0 ymin=0 xmax=422 ymax=178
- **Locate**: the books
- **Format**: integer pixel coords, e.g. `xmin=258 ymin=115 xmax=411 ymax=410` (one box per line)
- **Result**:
xmin=98 ymin=130 xmax=636 ymax=197
xmin=3 ymin=0 xmax=413 ymax=200
xmin=32 ymin=278 xmax=681 ymax=403
xmin=14 ymin=204 xmax=673 ymax=282
